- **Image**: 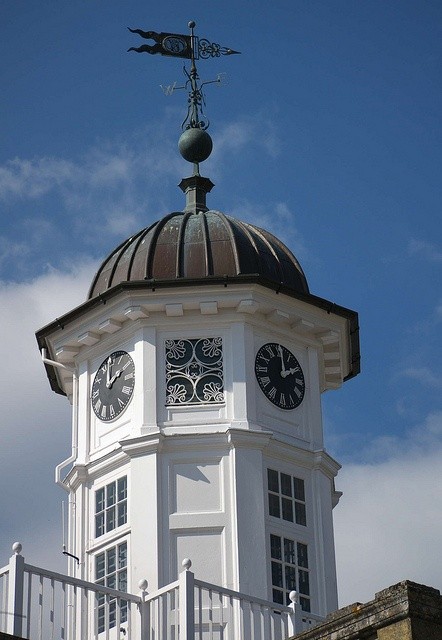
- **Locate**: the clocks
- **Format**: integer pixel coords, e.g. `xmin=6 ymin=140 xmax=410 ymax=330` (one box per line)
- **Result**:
xmin=89 ymin=350 xmax=136 ymax=424
xmin=255 ymin=343 xmax=307 ymax=409
